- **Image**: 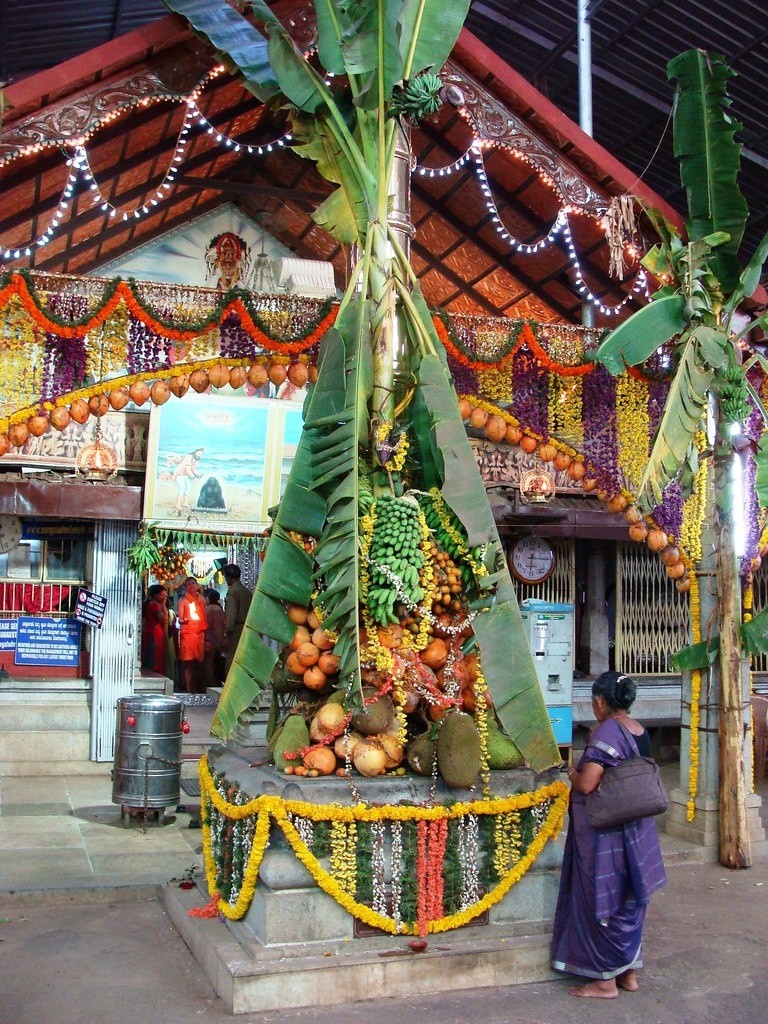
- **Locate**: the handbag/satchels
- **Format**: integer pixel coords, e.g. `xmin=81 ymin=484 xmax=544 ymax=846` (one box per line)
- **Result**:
xmin=583 ymin=719 xmax=667 ymax=827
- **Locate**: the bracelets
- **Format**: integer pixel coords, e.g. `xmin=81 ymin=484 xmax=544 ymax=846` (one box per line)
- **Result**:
xmin=567 ymin=769 xmax=575 ymax=780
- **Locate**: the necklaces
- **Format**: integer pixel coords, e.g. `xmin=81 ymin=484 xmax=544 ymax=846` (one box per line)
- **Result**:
xmin=613 ymin=715 xmax=625 ymax=720
xmin=142 ymin=585 xmax=169 ymax=675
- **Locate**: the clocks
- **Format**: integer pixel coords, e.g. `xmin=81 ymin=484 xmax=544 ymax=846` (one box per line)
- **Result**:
xmin=0 ymin=514 xmax=24 ymax=555
xmin=507 ymin=533 xmax=557 ymax=585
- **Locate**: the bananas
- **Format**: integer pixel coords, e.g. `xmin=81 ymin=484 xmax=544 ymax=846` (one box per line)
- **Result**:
xmin=389 ymin=72 xmax=443 ymax=118
xmin=356 ymin=489 xmax=483 ymax=628
xmin=712 ymin=362 xmax=754 ymax=421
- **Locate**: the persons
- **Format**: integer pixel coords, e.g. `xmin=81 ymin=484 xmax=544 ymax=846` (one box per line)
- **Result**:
xmin=551 ymin=672 xmax=668 ymax=999
xmin=143 ymin=586 xmax=224 ymax=687
xmin=178 ymin=577 xmax=208 ymax=693
xmin=222 ymin=564 xmax=253 ymax=657
